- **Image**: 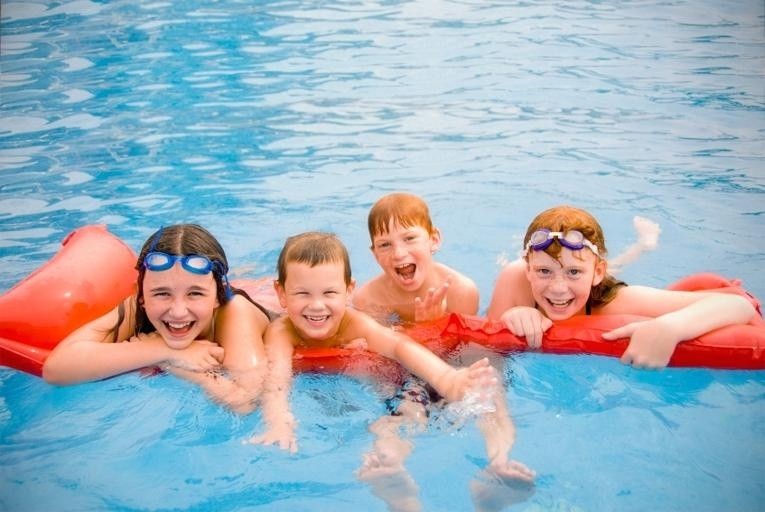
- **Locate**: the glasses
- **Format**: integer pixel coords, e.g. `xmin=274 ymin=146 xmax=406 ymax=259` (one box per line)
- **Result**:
xmin=143 ymin=227 xmax=231 ymax=300
xmin=523 ymin=229 xmax=598 ymax=258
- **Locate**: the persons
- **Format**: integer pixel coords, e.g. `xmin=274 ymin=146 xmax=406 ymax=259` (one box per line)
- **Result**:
xmin=488 ymin=206 xmax=758 ymax=372
xmin=42 ymin=223 xmax=285 ymax=414
xmin=348 ymin=195 xmax=539 ymax=511
xmin=242 ymin=232 xmax=500 ymax=456
